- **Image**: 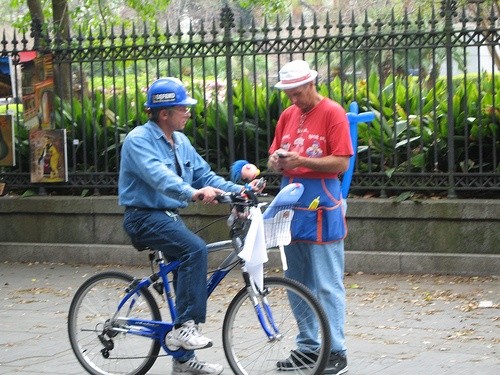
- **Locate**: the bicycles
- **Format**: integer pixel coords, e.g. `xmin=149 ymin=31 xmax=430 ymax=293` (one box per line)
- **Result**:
xmin=67 ymin=160 xmax=331 ymax=374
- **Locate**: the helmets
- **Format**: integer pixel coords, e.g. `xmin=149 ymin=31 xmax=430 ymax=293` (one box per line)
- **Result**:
xmin=143 ymin=77 xmax=197 ymax=108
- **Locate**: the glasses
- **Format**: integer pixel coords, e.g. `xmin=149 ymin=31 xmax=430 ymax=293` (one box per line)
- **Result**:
xmin=167 ymin=107 xmax=192 ymax=118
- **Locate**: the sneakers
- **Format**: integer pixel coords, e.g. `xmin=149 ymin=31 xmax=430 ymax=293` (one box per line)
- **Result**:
xmin=165 ymin=320 xmax=213 ymax=352
xmin=171 ymin=354 xmax=224 ymax=375
xmin=314 ymin=351 xmax=349 ymax=375
xmin=276 ymin=348 xmax=322 ymax=370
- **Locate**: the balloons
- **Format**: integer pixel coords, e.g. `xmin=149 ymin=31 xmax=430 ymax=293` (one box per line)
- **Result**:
xmin=339 ymin=102 xmax=375 ymax=197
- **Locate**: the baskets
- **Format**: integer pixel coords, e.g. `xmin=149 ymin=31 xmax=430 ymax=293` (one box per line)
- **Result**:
xmin=229 ymin=201 xmax=300 ymax=253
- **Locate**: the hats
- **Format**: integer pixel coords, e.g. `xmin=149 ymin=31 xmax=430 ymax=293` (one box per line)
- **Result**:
xmin=274 ymin=59 xmax=318 ymax=89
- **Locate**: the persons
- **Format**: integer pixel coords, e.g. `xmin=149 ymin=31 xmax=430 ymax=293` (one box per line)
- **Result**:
xmin=118 ymin=78 xmax=266 ymax=375
xmin=266 ymin=59 xmax=354 ymax=375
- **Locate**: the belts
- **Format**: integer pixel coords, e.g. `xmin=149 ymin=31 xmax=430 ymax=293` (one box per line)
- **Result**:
xmin=127 ymin=206 xmax=181 ymax=213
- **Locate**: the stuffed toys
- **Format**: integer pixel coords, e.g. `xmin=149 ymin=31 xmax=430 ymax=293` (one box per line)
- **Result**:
xmin=229 ymin=160 xmax=258 ymax=184
